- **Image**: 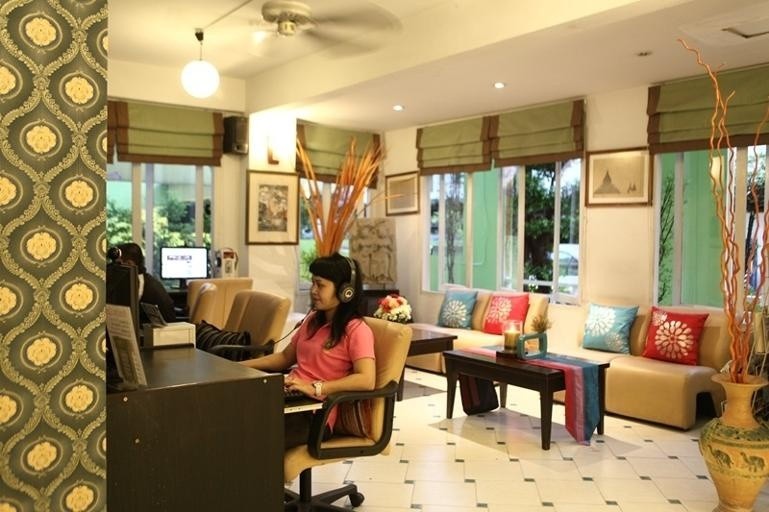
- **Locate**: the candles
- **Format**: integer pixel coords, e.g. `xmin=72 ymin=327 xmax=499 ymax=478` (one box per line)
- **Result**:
xmin=505 ymin=330 xmax=521 ymax=347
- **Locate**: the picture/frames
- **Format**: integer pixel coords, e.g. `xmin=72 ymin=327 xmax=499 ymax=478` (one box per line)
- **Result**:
xmin=384 ymin=170 xmax=421 ymax=216
xmin=245 ymin=169 xmax=301 ymax=247
xmin=584 ymin=145 xmax=654 ymax=208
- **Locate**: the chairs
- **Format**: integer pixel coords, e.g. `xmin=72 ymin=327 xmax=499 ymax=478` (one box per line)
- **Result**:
xmin=208 ymin=291 xmax=291 ymax=362
xmin=285 ymin=316 xmax=413 ymax=511
xmin=185 ymin=277 xmax=253 ymax=329
xmin=191 ymin=282 xmax=218 ymax=329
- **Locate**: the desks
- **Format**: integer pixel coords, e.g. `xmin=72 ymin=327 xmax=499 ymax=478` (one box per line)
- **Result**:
xmin=106 ymin=344 xmax=285 ymax=511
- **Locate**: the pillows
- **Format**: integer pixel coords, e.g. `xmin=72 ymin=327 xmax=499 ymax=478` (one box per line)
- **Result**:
xmin=437 ymin=291 xmax=478 ymax=329
xmin=335 ymin=398 xmax=372 ymax=438
xmin=582 ymin=302 xmax=640 ymax=355
xmin=195 ymin=322 xmax=249 ymax=362
xmin=641 ymin=306 xmax=710 ymax=366
xmin=482 ymin=294 xmax=530 ymax=334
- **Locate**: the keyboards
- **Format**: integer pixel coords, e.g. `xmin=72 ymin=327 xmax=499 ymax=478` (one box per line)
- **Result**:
xmin=284 ymin=385 xmax=304 ymax=401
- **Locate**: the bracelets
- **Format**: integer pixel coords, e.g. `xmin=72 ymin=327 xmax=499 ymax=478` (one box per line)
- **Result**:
xmin=313 ymin=381 xmax=323 ymax=397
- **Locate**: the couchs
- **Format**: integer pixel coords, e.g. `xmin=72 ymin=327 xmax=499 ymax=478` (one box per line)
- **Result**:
xmin=553 ymin=300 xmax=733 ymax=431
xmin=405 ymin=288 xmax=550 ymax=377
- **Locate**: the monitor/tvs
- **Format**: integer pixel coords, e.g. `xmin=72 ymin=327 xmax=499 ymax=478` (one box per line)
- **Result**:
xmin=106 ymin=263 xmax=141 ymax=355
xmin=160 ymin=246 xmax=208 ymax=289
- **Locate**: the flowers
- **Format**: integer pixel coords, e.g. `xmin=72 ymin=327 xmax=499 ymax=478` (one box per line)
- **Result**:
xmin=375 ymin=294 xmax=411 ymax=322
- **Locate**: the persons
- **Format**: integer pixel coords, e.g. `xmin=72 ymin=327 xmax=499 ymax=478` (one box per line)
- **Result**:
xmin=118 ymin=242 xmax=176 ymax=322
xmin=236 ymin=252 xmax=377 ymax=451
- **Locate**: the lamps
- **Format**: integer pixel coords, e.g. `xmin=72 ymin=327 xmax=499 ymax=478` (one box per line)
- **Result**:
xmin=182 ymin=31 xmax=221 ymax=99
xmin=267 ymin=145 xmax=281 ymax=164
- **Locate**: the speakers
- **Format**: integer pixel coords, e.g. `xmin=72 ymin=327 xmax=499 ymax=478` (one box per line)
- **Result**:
xmin=223 ymin=115 xmax=250 ymax=155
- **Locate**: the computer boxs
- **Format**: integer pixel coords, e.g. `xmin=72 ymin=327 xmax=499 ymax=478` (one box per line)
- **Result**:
xmin=215 ymin=248 xmax=238 ymax=278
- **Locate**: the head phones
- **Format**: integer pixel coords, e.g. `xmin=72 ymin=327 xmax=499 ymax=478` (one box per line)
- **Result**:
xmin=338 ymin=256 xmax=357 ymax=303
xmin=214 ymin=246 xmax=239 ymax=270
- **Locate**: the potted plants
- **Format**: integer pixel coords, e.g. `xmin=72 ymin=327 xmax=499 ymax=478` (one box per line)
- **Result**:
xmin=676 ymin=35 xmax=768 ymax=510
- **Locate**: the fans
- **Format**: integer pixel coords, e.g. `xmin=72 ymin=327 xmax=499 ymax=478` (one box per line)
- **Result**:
xmin=242 ymin=1 xmax=402 ymax=64
